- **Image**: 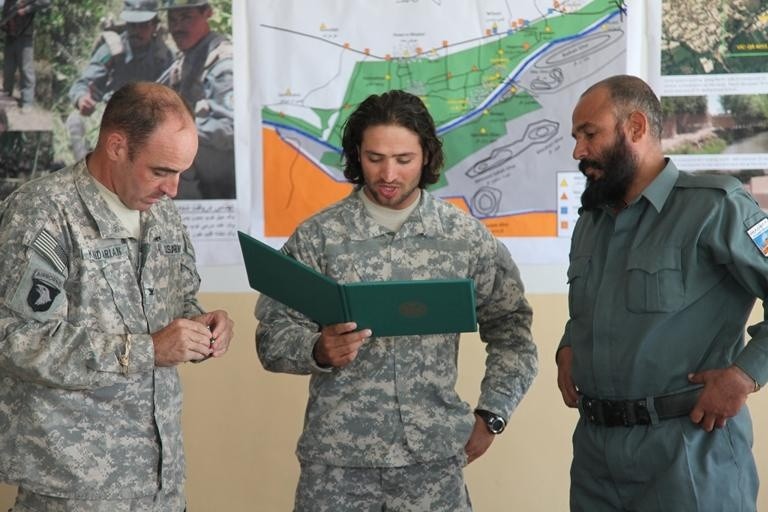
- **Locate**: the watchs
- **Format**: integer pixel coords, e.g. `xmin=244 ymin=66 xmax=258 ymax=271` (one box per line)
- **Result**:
xmin=475 ymin=409 xmax=506 ymax=435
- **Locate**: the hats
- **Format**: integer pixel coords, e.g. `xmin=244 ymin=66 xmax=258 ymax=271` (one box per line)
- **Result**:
xmin=155 ymin=0 xmax=207 ymax=10
xmin=119 ymin=0 xmax=159 ymax=23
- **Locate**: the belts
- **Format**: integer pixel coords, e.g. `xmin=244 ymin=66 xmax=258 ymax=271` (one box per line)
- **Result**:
xmin=576 ymin=388 xmax=707 ymax=427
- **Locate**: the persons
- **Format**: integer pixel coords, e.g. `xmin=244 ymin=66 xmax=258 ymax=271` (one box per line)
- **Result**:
xmin=554 ymin=74 xmax=768 ymax=512
xmin=0 ymin=81 xmax=234 ymax=512
xmin=149 ymin=0 xmax=234 ymax=199
xmin=66 ymin=0 xmax=173 ymax=167
xmin=252 ymin=90 xmax=539 ymax=512
xmin=0 ymin=0 xmax=52 ymax=116
